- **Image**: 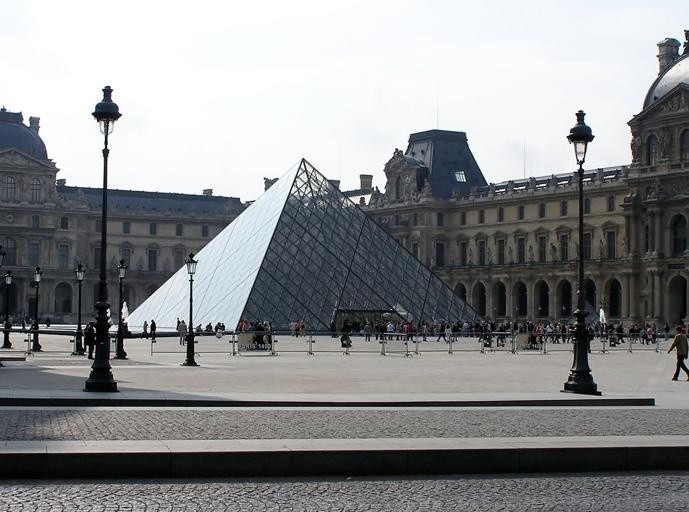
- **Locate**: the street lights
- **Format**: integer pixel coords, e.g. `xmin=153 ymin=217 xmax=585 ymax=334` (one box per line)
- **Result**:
xmin=113 ymin=256 xmax=130 ymax=360
xmin=29 ymin=262 xmax=46 ymax=351
xmin=1 ymin=269 xmax=14 ymax=348
xmin=561 ymin=105 xmax=601 ymax=396
xmin=77 ymin=86 xmax=132 ymax=397
xmin=71 ymin=260 xmax=86 ymax=354
xmin=180 ymin=252 xmax=200 ymax=367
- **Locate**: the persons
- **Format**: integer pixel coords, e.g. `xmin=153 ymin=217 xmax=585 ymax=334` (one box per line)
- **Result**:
xmin=20 ymin=316 xmax=51 ymax=332
xmin=585 ymin=321 xmax=688 ymax=352
xmin=120 ymin=319 xmax=128 ymax=337
xmin=332 ymin=318 xmax=430 ymax=341
xmin=176 ymin=317 xmax=225 ymax=346
xmin=668 ymin=327 xmax=689 ymax=382
xmin=289 ymin=319 xmax=307 ymax=338
xmin=83 ymin=322 xmax=96 ymax=360
xmin=431 ymin=320 xmax=511 ymax=347
xmin=141 ymin=320 xmax=156 ymax=341
xmin=512 ymin=320 xmax=577 ymax=349
xmin=238 ymin=318 xmax=272 ymax=350
